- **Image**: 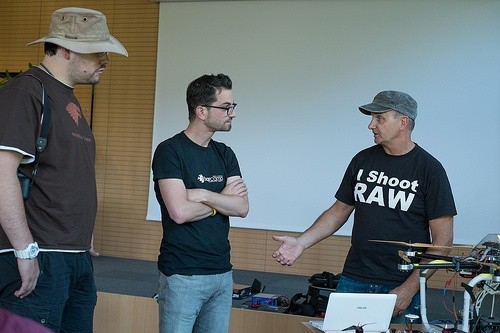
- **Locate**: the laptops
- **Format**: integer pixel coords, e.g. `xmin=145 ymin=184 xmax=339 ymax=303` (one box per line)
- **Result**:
xmin=310 ymin=293 xmax=398 ymax=333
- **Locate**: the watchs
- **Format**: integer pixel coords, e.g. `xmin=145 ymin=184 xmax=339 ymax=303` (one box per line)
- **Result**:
xmin=14 ymin=241 xmax=40 ymax=259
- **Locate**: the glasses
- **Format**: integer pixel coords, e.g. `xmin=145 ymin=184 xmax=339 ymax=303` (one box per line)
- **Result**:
xmin=97 ymin=52 xmax=109 ymax=56
xmin=202 ymin=103 xmax=237 ymax=115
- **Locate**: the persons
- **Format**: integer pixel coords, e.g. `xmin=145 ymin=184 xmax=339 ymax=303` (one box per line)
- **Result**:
xmin=152 ymin=73 xmax=250 ymax=333
xmin=0 ymin=7 xmax=129 ymax=333
xmin=272 ymin=90 xmax=458 ymax=324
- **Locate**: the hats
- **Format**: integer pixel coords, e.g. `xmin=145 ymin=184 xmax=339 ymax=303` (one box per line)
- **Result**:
xmin=25 ymin=7 xmax=130 ymax=57
xmin=358 ymin=90 xmax=418 ymax=121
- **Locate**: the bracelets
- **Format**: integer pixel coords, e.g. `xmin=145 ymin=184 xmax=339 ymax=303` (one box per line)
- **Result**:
xmin=212 ymin=208 xmax=217 ymax=215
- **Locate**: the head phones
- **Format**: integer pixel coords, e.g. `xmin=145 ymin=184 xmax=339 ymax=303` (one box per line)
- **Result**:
xmin=291 ymin=293 xmax=315 ymax=316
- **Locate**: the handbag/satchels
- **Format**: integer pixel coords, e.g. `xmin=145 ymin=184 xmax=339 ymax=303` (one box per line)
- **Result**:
xmin=308 ymin=271 xmax=342 ymax=289
xmin=14 ymin=73 xmax=50 ymax=199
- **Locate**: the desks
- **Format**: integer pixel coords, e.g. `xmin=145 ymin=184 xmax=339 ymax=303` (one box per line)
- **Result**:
xmin=300 ymin=320 xmax=500 ymax=333
